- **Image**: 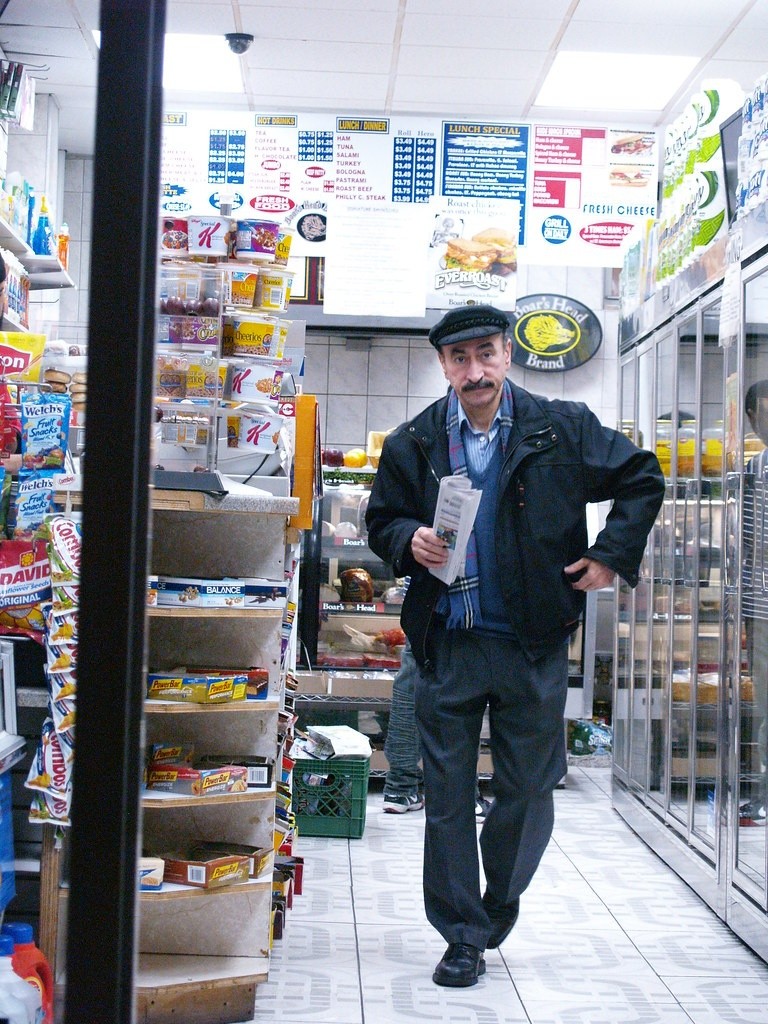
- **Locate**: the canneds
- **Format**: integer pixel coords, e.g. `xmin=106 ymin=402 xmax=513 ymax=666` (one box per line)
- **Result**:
xmin=621 ymin=418 xmax=722 ymax=479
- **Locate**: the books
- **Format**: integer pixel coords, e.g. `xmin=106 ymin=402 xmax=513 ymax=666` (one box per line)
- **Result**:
xmin=429 ymin=475 xmax=483 ymax=586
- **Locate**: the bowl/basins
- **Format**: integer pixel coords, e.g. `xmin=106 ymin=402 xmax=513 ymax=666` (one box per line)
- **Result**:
xmin=157 ymin=215 xmax=291 ymax=454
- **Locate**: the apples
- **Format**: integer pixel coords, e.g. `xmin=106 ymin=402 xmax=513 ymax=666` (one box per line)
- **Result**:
xmin=321 ymin=447 xmax=344 ymax=467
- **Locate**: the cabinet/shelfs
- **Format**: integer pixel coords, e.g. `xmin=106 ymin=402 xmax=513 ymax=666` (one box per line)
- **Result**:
xmin=0 ymin=39 xmax=93 ymax=1023
xmin=48 ymin=487 xmax=302 ymax=1023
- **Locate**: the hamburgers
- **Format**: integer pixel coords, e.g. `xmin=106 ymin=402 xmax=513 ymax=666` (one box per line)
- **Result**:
xmin=445 ymin=229 xmax=517 ymax=273
xmin=612 ymin=135 xmax=647 ymax=154
xmin=609 ymin=165 xmax=647 ymax=186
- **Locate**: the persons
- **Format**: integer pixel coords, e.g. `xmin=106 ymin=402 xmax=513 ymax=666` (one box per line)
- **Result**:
xmin=364 ymin=303 xmax=665 ymax=987
xmin=382 ymin=576 xmax=493 ymax=824
xmin=741 ymin=378 xmax=768 ymax=827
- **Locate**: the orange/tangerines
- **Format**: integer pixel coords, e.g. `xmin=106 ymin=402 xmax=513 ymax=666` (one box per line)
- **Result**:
xmin=343 ymin=448 xmax=368 ymax=468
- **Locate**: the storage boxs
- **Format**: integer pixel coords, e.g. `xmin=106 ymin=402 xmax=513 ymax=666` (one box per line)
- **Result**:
xmin=291 ymin=756 xmax=371 ymax=838
xmin=673 ymin=749 xmax=718 ymax=777
xmin=138 ymin=741 xmax=276 ymax=795
xmin=294 ymin=670 xmax=395 ymax=771
xmin=138 ymin=839 xmax=274 ymax=891
xmin=145 ymin=662 xmax=270 ymax=705
xmin=147 ymin=572 xmax=291 ymax=607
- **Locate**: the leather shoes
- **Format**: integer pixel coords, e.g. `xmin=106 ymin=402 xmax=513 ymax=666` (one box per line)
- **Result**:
xmin=432 ymin=943 xmax=487 ymax=987
xmin=479 ymin=883 xmax=520 ymax=949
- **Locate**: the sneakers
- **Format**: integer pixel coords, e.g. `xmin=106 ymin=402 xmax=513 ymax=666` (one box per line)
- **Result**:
xmin=383 ymin=792 xmax=424 ymax=814
xmin=736 ymin=800 xmax=768 ymax=827
xmin=473 ymin=795 xmax=494 ymax=823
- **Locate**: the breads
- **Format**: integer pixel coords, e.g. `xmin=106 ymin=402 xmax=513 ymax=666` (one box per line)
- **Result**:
xmin=669 ymin=678 xmax=755 ymax=706
xmin=40 ymin=369 xmax=87 ymax=411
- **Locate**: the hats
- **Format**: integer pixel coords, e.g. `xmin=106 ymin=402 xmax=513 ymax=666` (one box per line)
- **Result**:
xmin=429 ymin=304 xmax=510 ymax=353
